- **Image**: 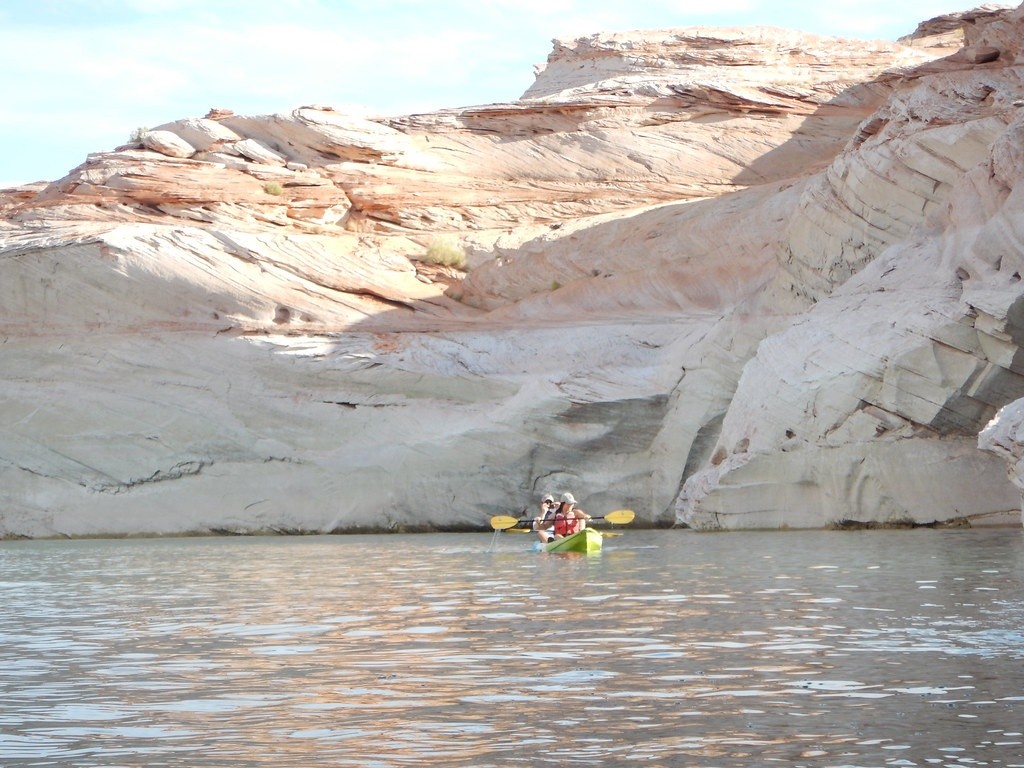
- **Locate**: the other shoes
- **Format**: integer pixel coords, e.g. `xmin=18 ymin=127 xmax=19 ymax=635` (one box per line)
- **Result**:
xmin=548 ymin=537 xmax=554 ymax=543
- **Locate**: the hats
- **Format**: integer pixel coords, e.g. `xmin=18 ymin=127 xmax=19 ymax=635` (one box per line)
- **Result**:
xmin=541 ymin=494 xmax=554 ymax=502
xmin=560 ymin=492 xmax=577 ymax=505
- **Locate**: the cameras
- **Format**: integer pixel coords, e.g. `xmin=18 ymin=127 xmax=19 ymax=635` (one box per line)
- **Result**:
xmin=547 ymin=505 xmax=554 ymax=509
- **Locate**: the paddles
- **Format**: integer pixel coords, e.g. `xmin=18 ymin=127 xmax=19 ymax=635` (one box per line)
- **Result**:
xmin=505 ymin=528 xmax=624 ymax=538
xmin=490 ymin=509 xmax=636 ymax=531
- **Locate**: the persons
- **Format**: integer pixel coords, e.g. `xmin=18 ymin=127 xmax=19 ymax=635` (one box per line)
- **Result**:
xmin=533 ymin=494 xmax=560 ymax=542
xmin=536 ymin=492 xmax=592 ymax=540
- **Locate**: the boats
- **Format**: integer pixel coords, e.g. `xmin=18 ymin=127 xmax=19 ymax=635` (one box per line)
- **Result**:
xmin=531 ymin=527 xmax=605 ymax=557
